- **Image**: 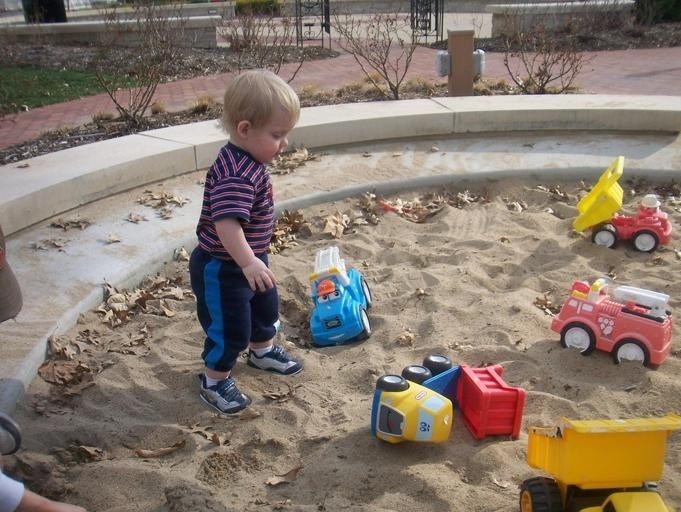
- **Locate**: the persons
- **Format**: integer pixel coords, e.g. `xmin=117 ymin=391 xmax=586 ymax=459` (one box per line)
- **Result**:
xmin=0 ymin=229 xmax=89 ymax=511
xmin=188 ymin=68 xmax=303 ymax=416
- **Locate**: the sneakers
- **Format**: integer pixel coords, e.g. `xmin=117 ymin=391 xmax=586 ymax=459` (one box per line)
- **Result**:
xmin=242 ymin=345 xmax=304 ymax=377
xmin=197 ymin=372 xmax=252 ymax=417
